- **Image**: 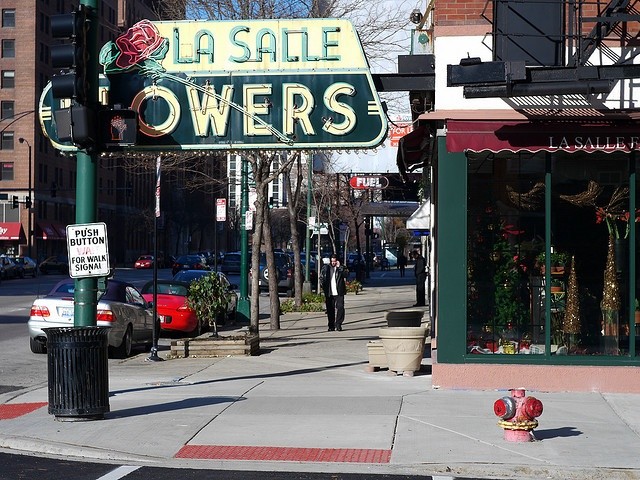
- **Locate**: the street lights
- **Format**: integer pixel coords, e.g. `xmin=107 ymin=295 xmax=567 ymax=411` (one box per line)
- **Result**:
xmin=18 ymin=138 xmax=32 ymax=259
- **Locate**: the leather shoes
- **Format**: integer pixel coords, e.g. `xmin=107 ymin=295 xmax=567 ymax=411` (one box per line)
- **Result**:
xmin=328 ymin=328 xmax=335 ymax=331
xmin=337 ymin=327 xmax=342 ymax=331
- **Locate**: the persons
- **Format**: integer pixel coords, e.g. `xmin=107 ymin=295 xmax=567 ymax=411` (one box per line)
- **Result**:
xmin=319 ymin=254 xmax=350 ymax=332
xmin=397 ymin=253 xmax=407 ymax=278
xmin=410 ymin=250 xmax=427 ymax=307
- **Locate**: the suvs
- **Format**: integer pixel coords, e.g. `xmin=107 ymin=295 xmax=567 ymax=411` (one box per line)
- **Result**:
xmin=247 ymin=251 xmax=294 ymax=297
xmin=220 ymin=252 xmax=249 ymax=275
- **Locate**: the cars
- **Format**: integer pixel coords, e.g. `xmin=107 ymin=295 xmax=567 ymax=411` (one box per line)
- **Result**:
xmin=170 ymin=270 xmax=238 ymax=325
xmin=140 ymin=278 xmax=210 ymax=336
xmin=134 ymin=255 xmax=154 ymax=269
xmin=196 ymin=251 xmax=214 ymax=266
xmin=27 ymin=278 xmax=161 ymax=359
xmin=172 ymin=254 xmax=212 ymax=277
xmin=0 ymin=257 xmax=18 ymax=280
xmin=298 ymin=250 xmax=382 ymax=290
xmin=38 ymin=256 xmax=68 ymax=275
xmin=210 ymin=250 xmax=226 ymax=265
xmin=273 ymin=248 xmax=285 ymax=254
xmin=10 ymin=257 xmax=38 ymax=279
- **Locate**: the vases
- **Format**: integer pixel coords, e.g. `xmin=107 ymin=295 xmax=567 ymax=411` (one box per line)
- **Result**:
xmin=615 ymin=240 xmax=628 ymax=271
xmin=379 ymin=326 xmax=429 ymax=373
xmin=368 ymin=339 xmax=388 ymax=372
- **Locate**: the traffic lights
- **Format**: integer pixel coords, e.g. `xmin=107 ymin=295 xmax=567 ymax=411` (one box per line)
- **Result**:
xmin=103 ymin=109 xmax=138 ymax=148
xmin=49 ymin=9 xmax=85 ymax=103
xmin=12 ymin=196 xmax=19 ymax=209
xmin=26 ymin=196 xmax=32 ymax=210
xmin=269 ymin=197 xmax=274 ymax=209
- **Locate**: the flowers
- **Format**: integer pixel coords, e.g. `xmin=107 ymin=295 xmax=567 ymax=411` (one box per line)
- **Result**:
xmin=596 ymin=205 xmax=640 ymax=239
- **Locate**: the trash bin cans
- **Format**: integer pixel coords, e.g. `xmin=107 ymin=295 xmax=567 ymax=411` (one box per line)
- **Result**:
xmin=41 ymin=326 xmax=112 ymax=421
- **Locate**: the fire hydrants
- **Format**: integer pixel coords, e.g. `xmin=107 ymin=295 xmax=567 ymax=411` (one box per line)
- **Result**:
xmin=494 ymin=387 xmax=542 ymax=442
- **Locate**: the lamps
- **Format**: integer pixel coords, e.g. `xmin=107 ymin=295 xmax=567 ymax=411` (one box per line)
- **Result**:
xmin=411 ymin=10 xmax=423 ymax=24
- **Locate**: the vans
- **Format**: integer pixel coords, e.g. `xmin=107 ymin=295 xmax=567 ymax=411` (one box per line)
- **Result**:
xmin=148 ymin=250 xmax=166 ymax=270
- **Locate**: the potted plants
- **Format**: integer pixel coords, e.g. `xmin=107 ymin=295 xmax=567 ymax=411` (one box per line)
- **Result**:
xmin=537 ymin=253 xmax=556 ymax=274
xmin=633 ymin=298 xmax=640 ymax=324
xmin=549 ymin=280 xmax=562 ymax=292
xmin=556 ymin=253 xmax=567 ymax=272
